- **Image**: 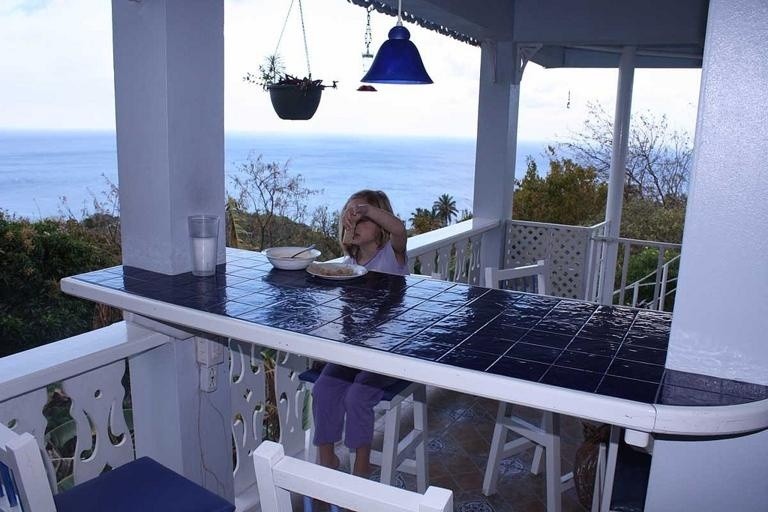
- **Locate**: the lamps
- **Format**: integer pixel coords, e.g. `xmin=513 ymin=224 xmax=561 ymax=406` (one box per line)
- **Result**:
xmin=361 ymin=0 xmax=434 ymax=84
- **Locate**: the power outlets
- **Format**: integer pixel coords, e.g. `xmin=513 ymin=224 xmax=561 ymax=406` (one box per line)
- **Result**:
xmin=200 ymin=364 xmax=217 ymax=392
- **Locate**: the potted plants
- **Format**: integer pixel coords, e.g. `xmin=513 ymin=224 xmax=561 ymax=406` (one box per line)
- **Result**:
xmin=243 ymin=55 xmax=339 ymax=119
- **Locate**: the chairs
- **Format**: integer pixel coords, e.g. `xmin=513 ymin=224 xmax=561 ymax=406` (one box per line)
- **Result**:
xmin=0 ymin=423 xmax=237 ymax=512
xmin=253 ymin=441 xmax=454 ymax=512
xmin=481 ymin=259 xmax=593 ymax=512
xmin=299 ymin=273 xmax=440 ymax=495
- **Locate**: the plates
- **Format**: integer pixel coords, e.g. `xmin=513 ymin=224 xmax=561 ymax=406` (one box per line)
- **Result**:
xmin=308 ymin=262 xmax=368 ymax=280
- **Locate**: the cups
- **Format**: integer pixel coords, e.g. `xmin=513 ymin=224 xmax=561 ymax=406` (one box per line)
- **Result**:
xmin=186 ymin=214 xmax=219 ymax=278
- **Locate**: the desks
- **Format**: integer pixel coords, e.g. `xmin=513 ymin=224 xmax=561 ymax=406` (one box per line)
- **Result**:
xmin=61 ymin=247 xmax=767 ymax=438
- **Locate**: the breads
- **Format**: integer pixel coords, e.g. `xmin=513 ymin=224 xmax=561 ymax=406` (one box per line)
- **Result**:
xmin=343 ymin=221 xmax=356 ymax=245
xmin=310 ymin=263 xmax=352 ymax=276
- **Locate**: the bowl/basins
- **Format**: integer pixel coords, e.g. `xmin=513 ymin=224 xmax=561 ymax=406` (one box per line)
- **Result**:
xmin=262 ymin=246 xmax=321 ymax=270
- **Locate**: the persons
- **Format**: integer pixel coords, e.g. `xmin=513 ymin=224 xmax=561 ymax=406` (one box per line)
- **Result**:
xmin=311 ymin=190 xmax=410 ymax=480
